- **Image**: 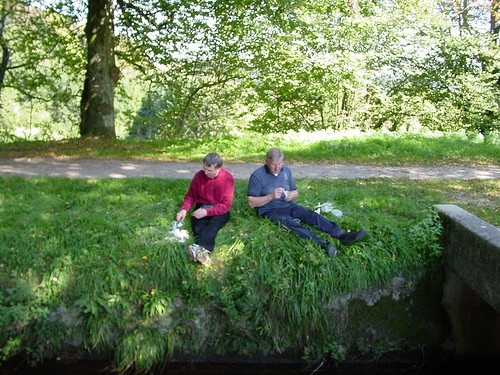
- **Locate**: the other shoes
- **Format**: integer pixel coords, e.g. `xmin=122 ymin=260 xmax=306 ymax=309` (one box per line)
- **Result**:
xmin=196 ymin=250 xmax=211 ymax=266
xmin=188 ymin=244 xmax=199 ymax=262
xmin=341 ymin=229 xmax=367 ymax=245
xmin=326 ymin=244 xmax=340 ymax=258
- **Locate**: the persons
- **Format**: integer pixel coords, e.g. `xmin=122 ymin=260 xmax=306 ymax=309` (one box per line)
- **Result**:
xmin=248 ymin=147 xmax=368 ymax=258
xmin=176 ymin=152 xmax=234 ymax=267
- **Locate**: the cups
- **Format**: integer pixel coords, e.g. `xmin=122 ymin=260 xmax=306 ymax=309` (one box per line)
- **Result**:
xmin=284 ymin=191 xmax=290 ymax=198
xmin=172 ymin=221 xmax=178 ymax=230
